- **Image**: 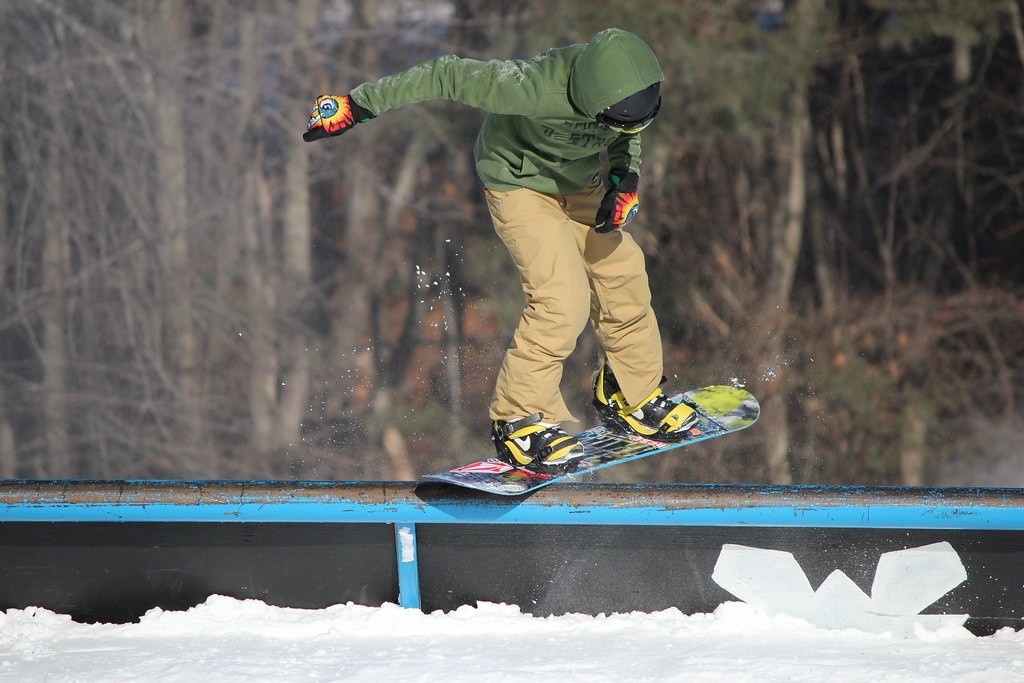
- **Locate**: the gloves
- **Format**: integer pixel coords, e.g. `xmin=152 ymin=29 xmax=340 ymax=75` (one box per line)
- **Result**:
xmin=595 ymin=172 xmax=639 ymax=233
xmin=303 ymin=95 xmax=377 ymax=142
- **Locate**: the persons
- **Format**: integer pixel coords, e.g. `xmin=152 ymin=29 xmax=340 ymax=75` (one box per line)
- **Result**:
xmin=303 ymin=27 xmax=699 ymax=473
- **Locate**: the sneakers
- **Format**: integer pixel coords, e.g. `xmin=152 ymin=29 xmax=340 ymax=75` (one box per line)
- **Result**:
xmin=490 ymin=419 xmax=583 ymax=466
xmin=591 ymin=360 xmax=698 ymax=435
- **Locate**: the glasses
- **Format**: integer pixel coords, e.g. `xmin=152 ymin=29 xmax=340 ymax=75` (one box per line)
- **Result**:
xmin=610 ymin=119 xmax=653 ymax=134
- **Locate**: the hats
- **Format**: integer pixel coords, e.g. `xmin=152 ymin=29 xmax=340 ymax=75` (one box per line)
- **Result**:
xmin=596 ymin=82 xmax=661 ymax=128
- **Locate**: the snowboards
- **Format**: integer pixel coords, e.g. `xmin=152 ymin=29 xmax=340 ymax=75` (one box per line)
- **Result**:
xmin=418 ymin=385 xmax=760 ymax=496
